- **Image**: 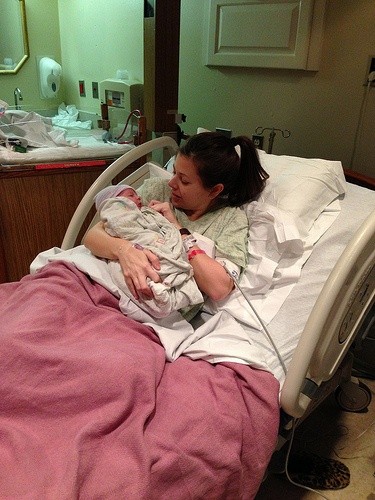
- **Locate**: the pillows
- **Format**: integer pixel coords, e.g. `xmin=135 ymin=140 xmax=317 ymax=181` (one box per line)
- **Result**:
xmin=166 ymin=128 xmax=346 ymax=268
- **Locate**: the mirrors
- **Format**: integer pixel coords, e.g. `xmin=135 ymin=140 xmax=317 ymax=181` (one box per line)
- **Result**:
xmin=0 ymin=0 xmax=30 ymax=76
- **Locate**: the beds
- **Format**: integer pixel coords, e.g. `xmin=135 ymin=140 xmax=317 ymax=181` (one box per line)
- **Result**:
xmin=0 ymin=137 xmax=375 ymax=500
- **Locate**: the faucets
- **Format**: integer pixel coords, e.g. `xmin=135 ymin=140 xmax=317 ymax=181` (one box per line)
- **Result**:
xmin=14 ymin=87 xmax=23 ymax=110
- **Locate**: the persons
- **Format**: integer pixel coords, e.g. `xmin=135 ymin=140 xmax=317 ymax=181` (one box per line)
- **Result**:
xmin=0 ymin=132 xmax=270 ymax=500
xmin=95 ymin=184 xmax=205 ymax=319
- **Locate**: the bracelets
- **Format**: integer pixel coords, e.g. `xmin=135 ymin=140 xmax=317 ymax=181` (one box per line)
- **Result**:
xmin=179 ymin=228 xmax=208 ymax=261
